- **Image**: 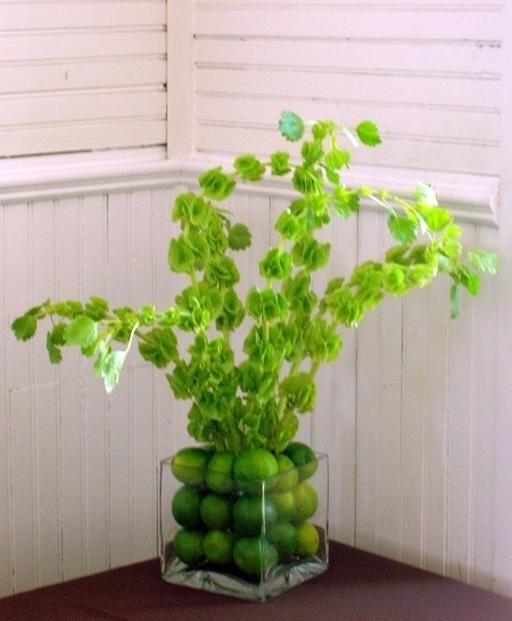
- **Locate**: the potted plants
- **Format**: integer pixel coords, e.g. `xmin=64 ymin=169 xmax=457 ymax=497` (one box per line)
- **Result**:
xmin=9 ymin=104 xmax=499 ymax=604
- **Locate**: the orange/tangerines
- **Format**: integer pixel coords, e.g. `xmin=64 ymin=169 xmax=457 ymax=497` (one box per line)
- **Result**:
xmin=171 ymin=442 xmax=320 ymax=575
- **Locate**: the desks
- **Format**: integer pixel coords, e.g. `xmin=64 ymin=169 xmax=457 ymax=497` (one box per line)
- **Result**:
xmin=1 ymin=540 xmax=512 ymax=621
